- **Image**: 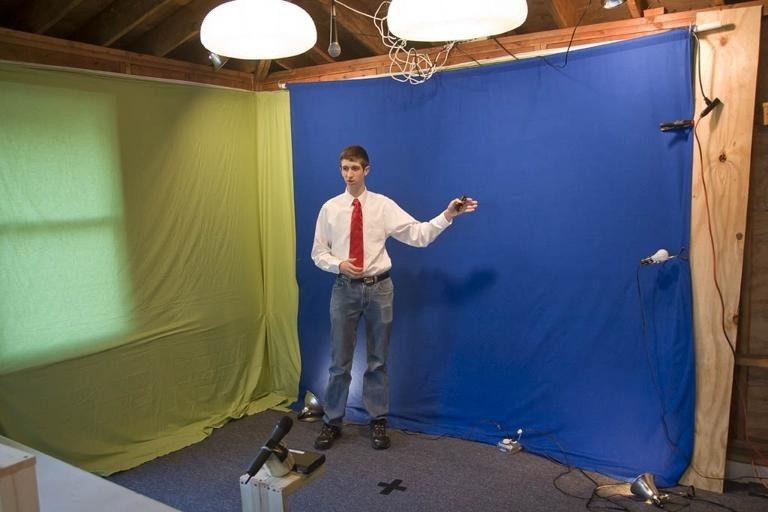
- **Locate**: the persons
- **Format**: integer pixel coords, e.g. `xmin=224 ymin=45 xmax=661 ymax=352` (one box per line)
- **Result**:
xmin=310 ymin=144 xmax=479 ymax=451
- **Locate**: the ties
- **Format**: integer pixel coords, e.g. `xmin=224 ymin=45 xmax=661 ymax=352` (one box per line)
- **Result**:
xmin=348 ymin=199 xmax=364 ymax=270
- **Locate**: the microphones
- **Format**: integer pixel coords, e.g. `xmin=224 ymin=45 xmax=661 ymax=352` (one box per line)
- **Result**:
xmin=248 ymin=416 xmax=293 ymax=477
xmin=328 ymin=1 xmax=342 ymax=57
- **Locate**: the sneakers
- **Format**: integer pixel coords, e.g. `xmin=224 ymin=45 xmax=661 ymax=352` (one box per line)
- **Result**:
xmin=313 ymin=423 xmax=340 ymax=450
xmin=371 ymin=419 xmax=392 ymax=450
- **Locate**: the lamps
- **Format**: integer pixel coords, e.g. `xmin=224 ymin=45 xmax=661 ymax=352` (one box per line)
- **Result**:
xmin=294 ymin=388 xmax=323 ymax=419
xmin=200 ymin=1 xmax=320 ymax=61
xmin=629 ymin=471 xmax=671 ymax=509
xmin=385 ymin=0 xmax=528 ymax=43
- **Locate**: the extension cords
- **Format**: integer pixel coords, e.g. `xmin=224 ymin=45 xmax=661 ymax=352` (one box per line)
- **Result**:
xmin=699 ymin=98 xmax=721 ymax=117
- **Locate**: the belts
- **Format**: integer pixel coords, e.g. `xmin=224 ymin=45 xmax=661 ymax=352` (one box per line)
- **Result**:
xmin=338 ymin=273 xmax=389 ymax=286
